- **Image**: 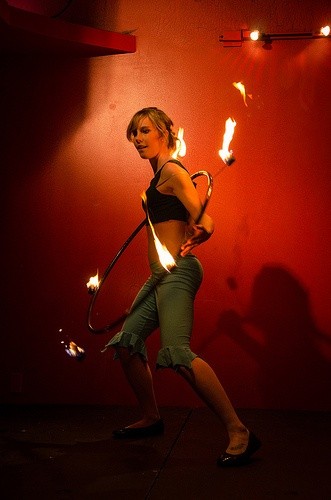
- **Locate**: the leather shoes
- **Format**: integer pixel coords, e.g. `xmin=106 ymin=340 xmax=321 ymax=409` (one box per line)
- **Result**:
xmin=112 ymin=418 xmax=165 ymax=440
xmin=217 ymin=430 xmax=261 ymax=467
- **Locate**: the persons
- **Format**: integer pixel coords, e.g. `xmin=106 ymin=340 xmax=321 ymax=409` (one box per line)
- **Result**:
xmin=105 ymin=107 xmax=262 ymax=468
